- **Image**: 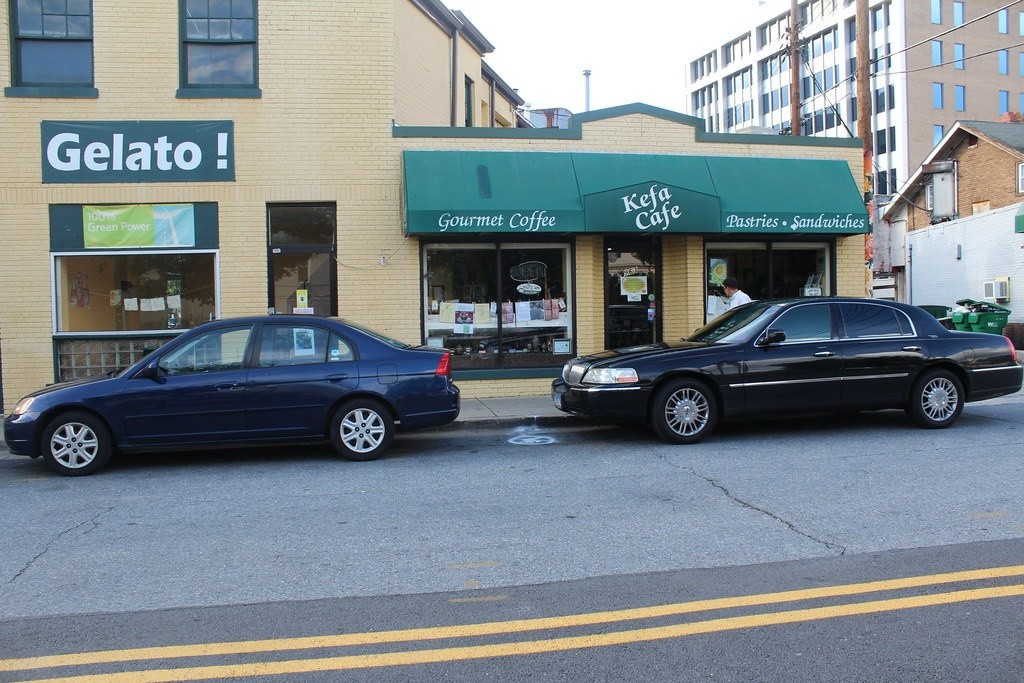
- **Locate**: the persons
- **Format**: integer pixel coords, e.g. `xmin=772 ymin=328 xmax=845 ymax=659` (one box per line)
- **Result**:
xmin=722 ymin=278 xmax=751 ymax=310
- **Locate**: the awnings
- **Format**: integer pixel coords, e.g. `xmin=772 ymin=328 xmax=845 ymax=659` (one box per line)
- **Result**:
xmin=402 ymin=151 xmax=870 ymax=236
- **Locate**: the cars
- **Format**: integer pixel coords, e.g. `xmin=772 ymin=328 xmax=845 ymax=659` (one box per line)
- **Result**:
xmin=2 ymin=314 xmax=462 ymax=477
xmin=551 ymin=294 xmax=1024 ymax=445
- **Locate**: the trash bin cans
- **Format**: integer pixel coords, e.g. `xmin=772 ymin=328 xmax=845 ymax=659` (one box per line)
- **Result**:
xmin=969 ymin=301 xmax=1011 ymax=336
xmin=953 ymin=299 xmax=976 ymax=332
xmin=916 ymin=305 xmax=952 ymax=320
xmin=936 ymin=316 xmax=956 ymax=330
